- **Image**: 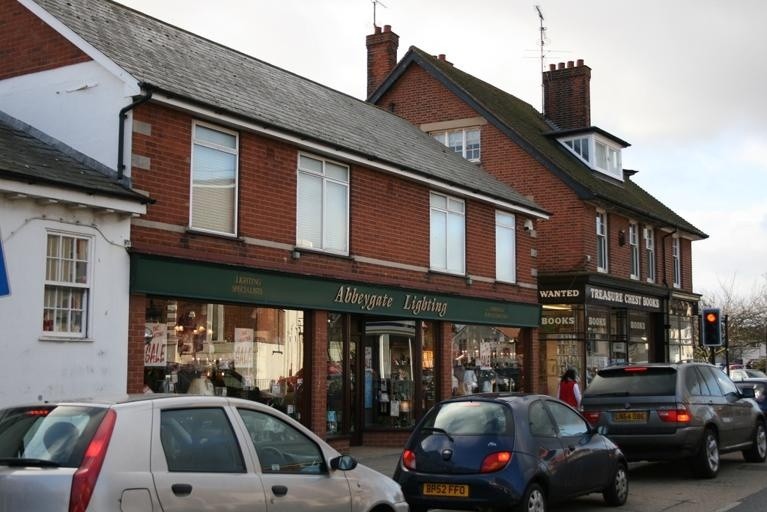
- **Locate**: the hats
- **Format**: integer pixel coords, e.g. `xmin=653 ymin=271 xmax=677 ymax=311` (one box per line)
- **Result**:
xmin=579 ymin=363 xmax=767 ymax=479
xmin=0 ymin=392 xmax=409 ymax=512
xmin=143 ymin=363 xmax=285 ymax=416
xmin=393 ymin=391 xmax=628 ymax=512
xmin=282 ymin=361 xmax=520 ymax=425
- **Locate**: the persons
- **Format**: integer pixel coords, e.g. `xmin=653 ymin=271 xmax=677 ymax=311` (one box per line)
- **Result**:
xmin=144 ymin=359 xmax=248 ymax=398
xmin=555 ymin=367 xmax=582 ymax=410
xmin=720 ymin=357 xmax=752 ymax=369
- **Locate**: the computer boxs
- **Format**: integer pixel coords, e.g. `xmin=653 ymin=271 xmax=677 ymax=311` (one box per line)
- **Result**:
xmin=524 ymin=227 xmax=532 ymax=232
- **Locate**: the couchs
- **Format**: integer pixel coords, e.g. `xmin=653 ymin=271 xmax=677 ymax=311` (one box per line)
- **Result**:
xmin=702 ymin=309 xmax=722 ymax=347
